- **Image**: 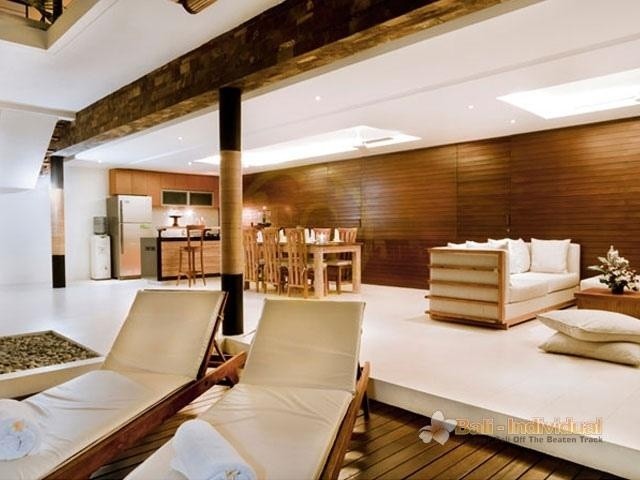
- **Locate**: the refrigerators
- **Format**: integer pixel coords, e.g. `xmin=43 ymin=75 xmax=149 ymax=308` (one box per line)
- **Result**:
xmin=106 ymin=195 xmax=154 ymax=280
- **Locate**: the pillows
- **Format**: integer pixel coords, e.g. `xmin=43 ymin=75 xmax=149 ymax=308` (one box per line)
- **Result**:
xmin=447 ymin=242 xmax=466 ymax=249
xmin=488 ymin=238 xmax=508 ymax=250
xmin=504 ymin=237 xmax=530 ymax=273
xmin=530 ymin=238 xmax=570 ymax=273
xmin=535 ymin=309 xmax=640 ymax=342
xmin=466 ymin=240 xmax=488 ymax=249
xmin=538 ymin=332 xmax=640 ymax=365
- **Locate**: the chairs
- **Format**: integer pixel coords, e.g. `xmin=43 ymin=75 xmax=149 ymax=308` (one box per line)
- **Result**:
xmin=242 ymin=228 xmax=264 ymax=293
xmin=284 ymin=228 xmax=329 ymax=299
xmin=324 ymin=227 xmax=358 ymax=295
xmin=0 ymin=289 xmax=248 ymax=480
xmin=124 ymin=298 xmax=371 ymax=479
xmin=312 ymin=227 xmax=332 ymax=259
xmin=262 ymin=227 xmax=288 ymax=295
xmin=176 ymin=225 xmax=206 ymax=287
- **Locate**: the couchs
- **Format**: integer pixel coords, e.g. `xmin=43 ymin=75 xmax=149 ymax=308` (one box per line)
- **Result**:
xmin=424 ymin=243 xmax=580 ymax=330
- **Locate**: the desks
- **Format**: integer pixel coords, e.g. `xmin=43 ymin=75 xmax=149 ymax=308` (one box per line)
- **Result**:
xmin=244 ymin=242 xmax=364 ymax=300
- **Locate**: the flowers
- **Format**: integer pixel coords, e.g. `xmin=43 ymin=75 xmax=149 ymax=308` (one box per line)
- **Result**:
xmin=586 ymin=244 xmax=640 ymax=292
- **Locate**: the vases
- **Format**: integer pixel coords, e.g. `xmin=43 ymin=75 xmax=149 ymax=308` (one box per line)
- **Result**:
xmin=609 ymin=276 xmax=625 ymax=293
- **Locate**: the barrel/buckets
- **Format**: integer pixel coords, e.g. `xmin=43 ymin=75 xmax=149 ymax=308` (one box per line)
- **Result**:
xmin=93 ymin=216 xmax=106 ymax=235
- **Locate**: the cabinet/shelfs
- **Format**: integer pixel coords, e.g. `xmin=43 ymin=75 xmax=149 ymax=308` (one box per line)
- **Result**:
xmin=109 ymin=169 xmax=132 ymax=196
xmin=147 ymin=172 xmax=161 ymax=207
xmin=132 ymin=171 xmax=147 ymax=195
